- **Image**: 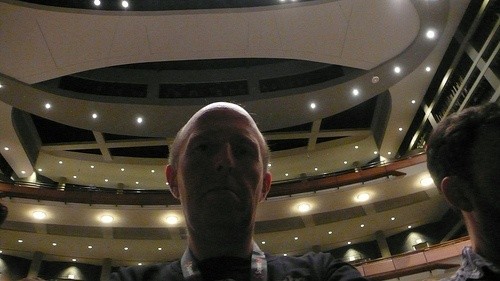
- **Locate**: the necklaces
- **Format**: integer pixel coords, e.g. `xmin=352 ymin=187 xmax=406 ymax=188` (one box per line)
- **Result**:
xmin=181 ymin=240 xmax=267 ymax=281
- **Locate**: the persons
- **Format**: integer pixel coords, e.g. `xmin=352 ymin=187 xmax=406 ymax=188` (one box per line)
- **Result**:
xmin=427 ymin=102 xmax=500 ymax=281
xmin=109 ymin=102 xmax=365 ymax=281
